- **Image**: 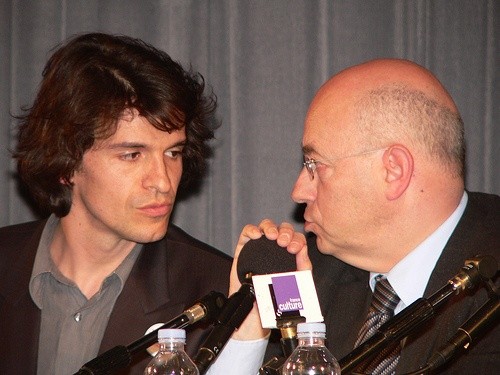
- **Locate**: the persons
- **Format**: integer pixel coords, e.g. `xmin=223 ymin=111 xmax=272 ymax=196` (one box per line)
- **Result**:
xmin=0 ymin=33 xmax=234 ymax=375
xmin=204 ymin=59 xmax=500 ymax=375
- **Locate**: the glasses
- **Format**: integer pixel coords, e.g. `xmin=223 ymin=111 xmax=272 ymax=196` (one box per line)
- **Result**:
xmin=301 ymin=146 xmax=390 ymax=174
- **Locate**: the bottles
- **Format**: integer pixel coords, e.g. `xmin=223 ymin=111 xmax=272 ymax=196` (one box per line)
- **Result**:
xmin=281 ymin=323 xmax=344 ymax=375
xmin=144 ymin=328 xmax=200 ymax=375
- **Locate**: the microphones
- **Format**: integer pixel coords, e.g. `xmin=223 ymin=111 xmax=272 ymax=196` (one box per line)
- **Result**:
xmin=74 ymin=235 xmax=500 ymax=375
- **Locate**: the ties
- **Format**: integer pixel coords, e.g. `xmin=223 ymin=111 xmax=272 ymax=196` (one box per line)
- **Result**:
xmin=349 ymin=276 xmax=403 ymax=375
